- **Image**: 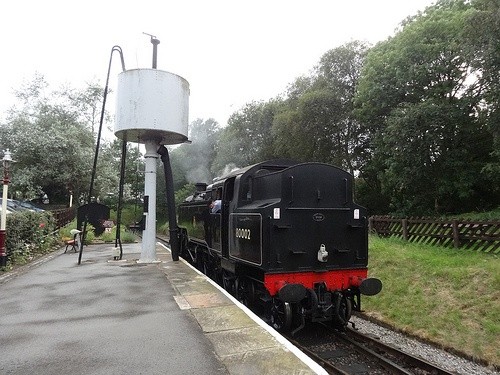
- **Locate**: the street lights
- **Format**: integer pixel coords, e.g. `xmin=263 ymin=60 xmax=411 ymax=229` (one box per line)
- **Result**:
xmin=0 ymin=148 xmax=16 ymax=270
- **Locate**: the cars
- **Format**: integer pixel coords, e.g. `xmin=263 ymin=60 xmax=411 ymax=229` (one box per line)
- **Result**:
xmin=0 ymin=198 xmax=47 ymax=216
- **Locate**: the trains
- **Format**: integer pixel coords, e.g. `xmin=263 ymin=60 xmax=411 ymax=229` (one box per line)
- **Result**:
xmin=178 ymin=157 xmax=384 ymax=333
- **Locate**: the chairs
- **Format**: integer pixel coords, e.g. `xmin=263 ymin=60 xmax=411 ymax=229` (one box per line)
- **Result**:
xmin=63 ymin=229 xmax=80 ymax=254
